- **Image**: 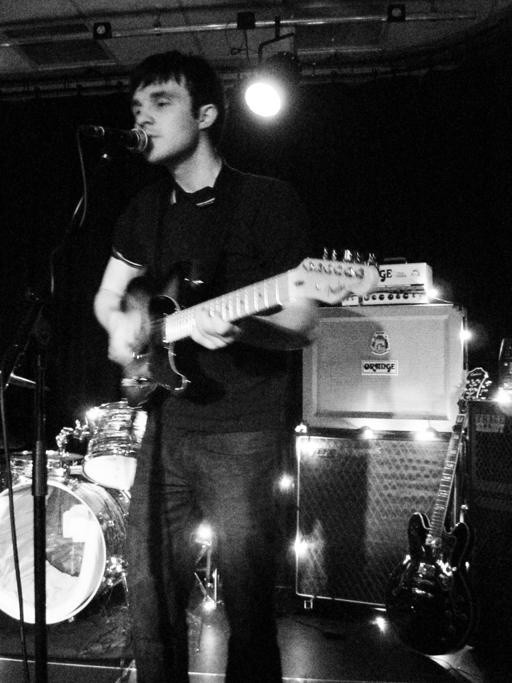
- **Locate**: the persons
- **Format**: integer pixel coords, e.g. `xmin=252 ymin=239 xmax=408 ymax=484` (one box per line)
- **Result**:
xmin=91 ymin=48 xmax=318 ymax=681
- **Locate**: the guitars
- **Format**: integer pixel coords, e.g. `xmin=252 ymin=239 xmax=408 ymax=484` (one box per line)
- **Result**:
xmin=122 ymin=248 xmax=380 ymax=406
xmin=384 ymin=368 xmax=491 ymax=656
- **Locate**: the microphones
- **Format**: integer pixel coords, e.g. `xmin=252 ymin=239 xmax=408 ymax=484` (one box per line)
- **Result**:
xmin=87 ymin=124 xmax=153 ymax=153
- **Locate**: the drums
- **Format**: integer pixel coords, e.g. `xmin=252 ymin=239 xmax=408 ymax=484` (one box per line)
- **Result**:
xmin=82 ymin=400 xmax=148 ymax=488
xmin=0 ymin=477 xmax=133 ymax=624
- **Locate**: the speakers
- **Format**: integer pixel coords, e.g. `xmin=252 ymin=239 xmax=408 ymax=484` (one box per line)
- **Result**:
xmin=294 ymin=433 xmax=460 ymax=610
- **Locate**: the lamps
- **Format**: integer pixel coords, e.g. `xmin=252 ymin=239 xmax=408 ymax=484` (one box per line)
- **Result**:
xmin=242 ymin=35 xmax=302 ymax=123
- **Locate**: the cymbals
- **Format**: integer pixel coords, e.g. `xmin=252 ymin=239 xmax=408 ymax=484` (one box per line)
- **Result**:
xmin=17 ymin=451 xmax=83 ymax=461
